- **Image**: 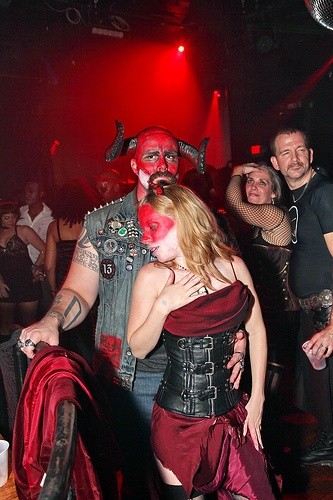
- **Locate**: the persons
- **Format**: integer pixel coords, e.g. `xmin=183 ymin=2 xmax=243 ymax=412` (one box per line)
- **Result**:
xmin=16 ymin=119 xmax=245 ymax=500
xmin=0 ymin=178 xmax=92 ymax=345
xmin=225 ymin=123 xmax=333 ymax=467
xmin=126 ymin=171 xmax=275 ymax=500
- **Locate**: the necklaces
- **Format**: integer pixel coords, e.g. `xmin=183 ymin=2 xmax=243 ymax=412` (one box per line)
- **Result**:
xmin=179 ymin=266 xmax=208 ymax=295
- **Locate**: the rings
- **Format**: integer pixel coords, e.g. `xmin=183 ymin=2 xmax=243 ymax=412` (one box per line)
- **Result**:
xmin=240 ymin=360 xmax=245 ymax=367
xmin=235 ymin=352 xmax=244 ymax=359
xmin=18 ymin=339 xmax=26 ymax=350
xmin=25 ymin=339 xmax=36 ymax=348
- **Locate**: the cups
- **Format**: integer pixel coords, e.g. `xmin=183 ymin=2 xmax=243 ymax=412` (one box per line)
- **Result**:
xmin=302 ymin=341 xmax=326 ymax=370
xmin=0 ymin=440 xmax=9 ymax=488
